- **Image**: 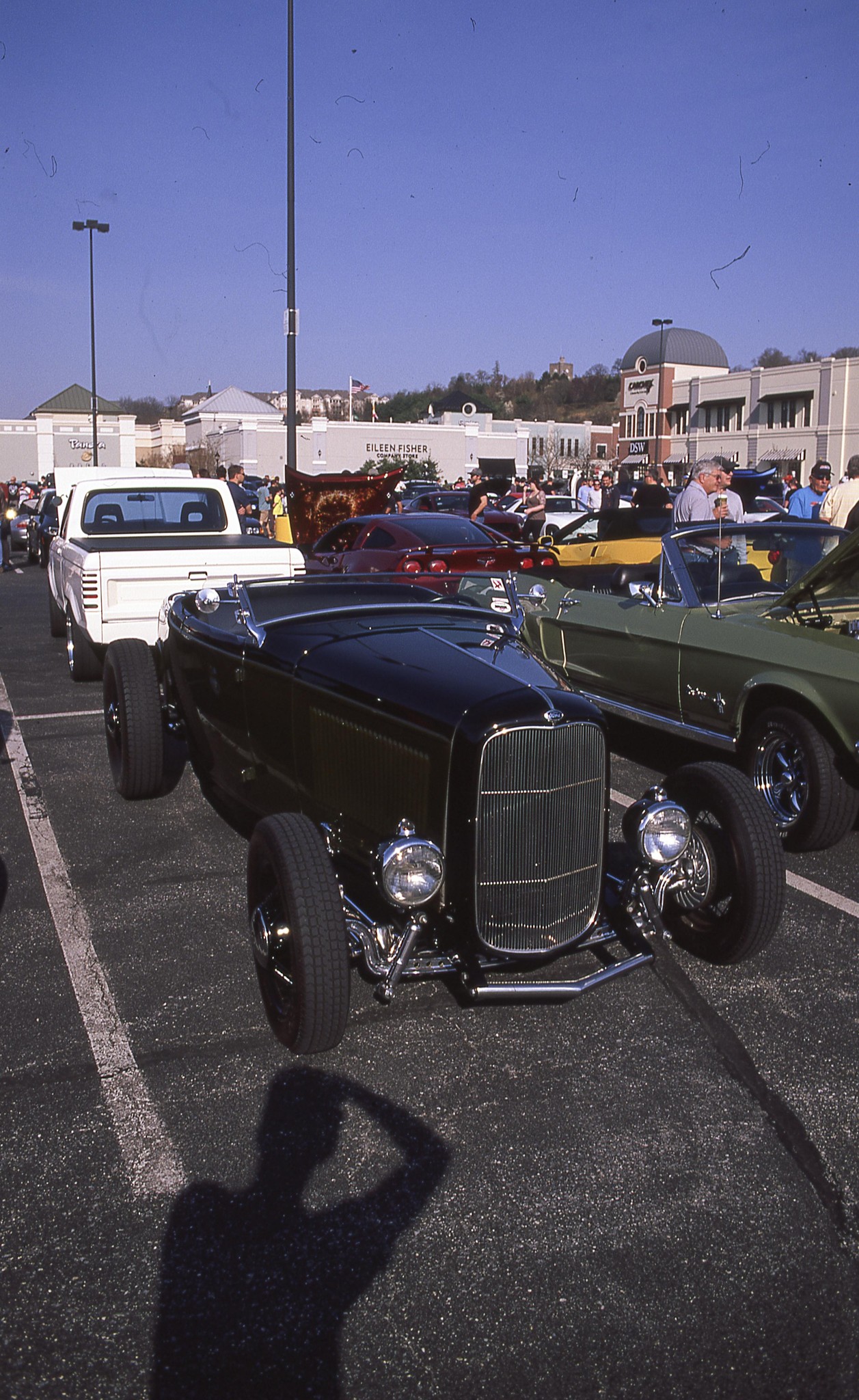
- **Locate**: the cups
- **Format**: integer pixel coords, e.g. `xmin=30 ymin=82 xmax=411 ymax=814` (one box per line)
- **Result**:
xmin=718 ymin=495 xmax=728 ymax=509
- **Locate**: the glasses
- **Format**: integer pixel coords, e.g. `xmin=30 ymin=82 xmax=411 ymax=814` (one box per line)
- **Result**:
xmin=811 ymin=473 xmax=830 ymax=480
xmin=237 ymin=472 xmax=245 ymax=475
xmin=720 ymin=468 xmax=733 ymax=474
xmin=703 ymin=472 xmax=721 ymax=480
xmin=594 ymin=484 xmax=600 ymax=485
xmin=645 ymin=475 xmax=651 ymax=478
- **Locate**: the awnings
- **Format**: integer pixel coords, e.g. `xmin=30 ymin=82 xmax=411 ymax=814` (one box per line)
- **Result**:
xmin=695 ymin=396 xmax=746 ymax=409
xmin=759 ymin=449 xmax=805 ymax=461
xmin=668 ymin=404 xmax=690 ymax=413
xmin=696 ymin=452 xmax=738 ymax=463
xmin=620 ymin=454 xmax=649 ymax=465
xmin=756 ymin=390 xmax=814 ymax=403
xmin=664 ymin=452 xmax=689 ymax=464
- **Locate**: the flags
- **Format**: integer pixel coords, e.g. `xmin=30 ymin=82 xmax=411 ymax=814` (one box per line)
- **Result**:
xmin=351 ymin=378 xmax=370 ymax=394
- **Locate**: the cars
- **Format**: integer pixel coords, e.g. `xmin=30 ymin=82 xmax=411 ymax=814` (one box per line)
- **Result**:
xmin=284 ymin=462 xmax=561 ymax=598
xmin=104 ymin=570 xmax=786 ymax=1051
xmin=505 ymin=479 xmax=798 ymax=599
xmin=455 ymin=522 xmax=859 ymax=855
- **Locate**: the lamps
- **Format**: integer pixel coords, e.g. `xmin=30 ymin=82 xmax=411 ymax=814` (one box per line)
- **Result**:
xmin=819 ymin=457 xmax=822 ymax=462
xmin=471 ymin=453 xmax=473 ymax=461
xmin=319 ymin=450 xmax=321 ymax=457
xmin=690 ymin=460 xmax=693 ymax=465
xmin=749 ymin=459 xmax=753 ymax=464
xmin=652 ymin=460 xmax=655 ymax=465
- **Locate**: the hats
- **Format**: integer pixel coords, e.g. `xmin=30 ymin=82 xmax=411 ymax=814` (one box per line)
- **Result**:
xmin=812 ymin=461 xmax=835 ymax=475
xmin=467 ymin=468 xmax=483 ymax=476
xmin=783 ymin=475 xmax=793 ymax=480
xmin=711 ymin=456 xmax=739 ymax=469
xmin=525 ymin=477 xmax=538 ymax=484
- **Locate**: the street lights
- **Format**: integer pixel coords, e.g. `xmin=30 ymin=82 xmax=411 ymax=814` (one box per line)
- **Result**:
xmin=72 ymin=220 xmax=111 ymax=467
xmin=652 ymin=318 xmax=673 ymax=482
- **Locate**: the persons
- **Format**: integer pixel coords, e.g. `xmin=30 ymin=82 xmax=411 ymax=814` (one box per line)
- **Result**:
xmin=189 ymin=464 xmax=252 ymax=535
xmin=0 ymin=476 xmax=48 ymax=573
xmin=467 ymin=468 xmax=488 ymax=523
xmin=578 ymin=474 xmax=620 ymax=510
xmin=258 ymin=474 xmax=288 ymax=540
xmin=672 ymin=456 xmax=749 ymax=564
xmin=510 ymin=476 xmax=556 ymax=495
xmin=778 ymin=455 xmax=859 ymax=589
xmin=442 ymin=477 xmax=474 ymax=492
xmin=385 ymin=480 xmax=407 ymax=514
xmin=522 ymin=478 xmax=547 ymax=542
xmin=630 ymin=469 xmax=674 ymax=509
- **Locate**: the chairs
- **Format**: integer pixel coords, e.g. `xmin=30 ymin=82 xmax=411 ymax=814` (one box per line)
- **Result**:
xmin=611 ymin=563 xmax=659 ymax=602
xmin=710 ymin=564 xmax=764 ymax=599
xmin=180 ymin=502 xmax=210 ymax=530
xmin=93 ymin=504 xmax=125 ymax=533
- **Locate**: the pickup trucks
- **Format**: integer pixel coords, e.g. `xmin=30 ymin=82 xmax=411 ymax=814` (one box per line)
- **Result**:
xmin=10 ymin=464 xmax=306 ymax=682
